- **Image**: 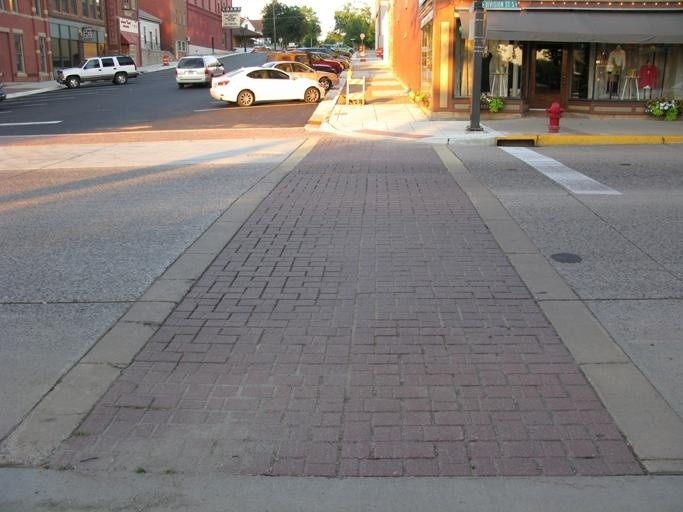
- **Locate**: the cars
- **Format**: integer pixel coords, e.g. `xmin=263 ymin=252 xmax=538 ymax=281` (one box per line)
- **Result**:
xmin=210 ymin=45 xmax=355 ymax=106
xmin=0 ymin=83 xmax=8 ymax=103
xmin=376 ymin=48 xmax=383 ymax=59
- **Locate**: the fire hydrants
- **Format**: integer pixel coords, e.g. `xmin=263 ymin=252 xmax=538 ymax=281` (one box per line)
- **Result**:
xmin=544 ymin=101 xmax=565 ymax=133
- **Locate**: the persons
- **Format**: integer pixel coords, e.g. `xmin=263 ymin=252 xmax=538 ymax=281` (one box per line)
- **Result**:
xmin=606 ymin=45 xmax=626 ymax=93
xmin=640 ymin=59 xmax=656 ymax=91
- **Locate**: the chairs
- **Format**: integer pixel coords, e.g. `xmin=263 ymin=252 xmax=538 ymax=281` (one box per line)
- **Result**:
xmin=346 ymin=76 xmax=366 ymax=113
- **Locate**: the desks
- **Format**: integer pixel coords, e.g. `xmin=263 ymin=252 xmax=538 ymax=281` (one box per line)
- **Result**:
xmin=491 ymin=73 xmax=510 ymax=98
xmin=620 ymin=75 xmax=642 ymax=100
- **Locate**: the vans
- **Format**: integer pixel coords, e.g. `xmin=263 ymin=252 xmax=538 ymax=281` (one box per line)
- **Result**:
xmin=175 ymin=55 xmax=226 ymax=88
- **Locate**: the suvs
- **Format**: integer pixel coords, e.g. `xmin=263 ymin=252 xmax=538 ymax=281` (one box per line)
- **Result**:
xmin=56 ymin=56 xmax=138 ymax=89
